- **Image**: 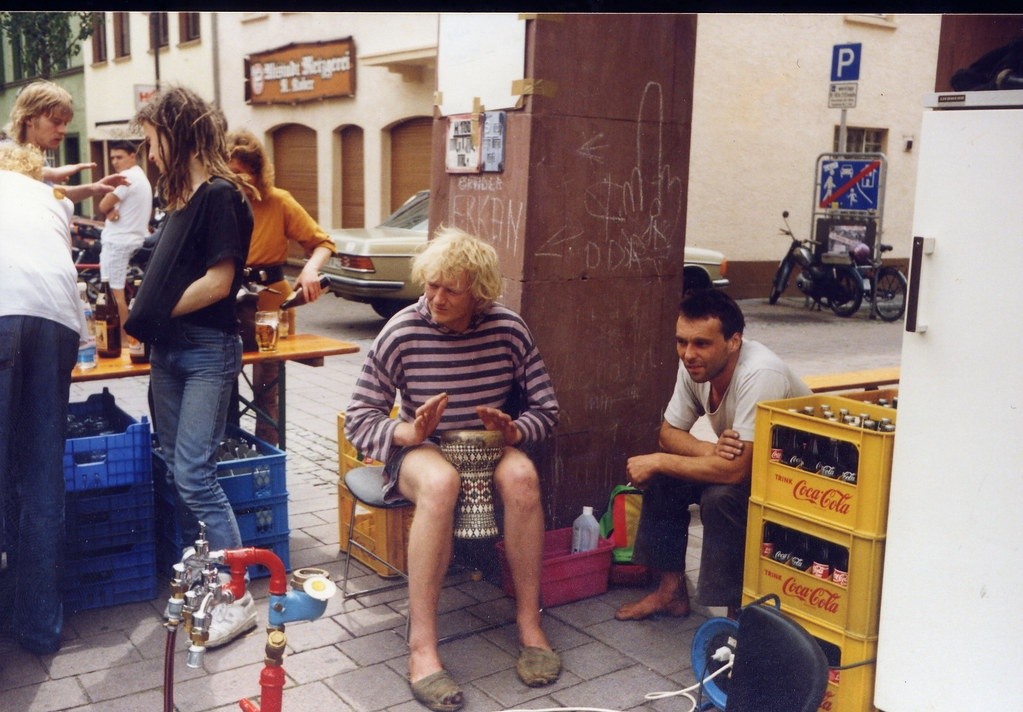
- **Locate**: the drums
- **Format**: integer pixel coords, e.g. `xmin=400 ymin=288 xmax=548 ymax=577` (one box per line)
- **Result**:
xmin=440 ymin=428 xmax=506 ymax=541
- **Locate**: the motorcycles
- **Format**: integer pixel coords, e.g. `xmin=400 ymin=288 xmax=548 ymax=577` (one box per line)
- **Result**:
xmin=768 ymin=211 xmax=863 ymax=316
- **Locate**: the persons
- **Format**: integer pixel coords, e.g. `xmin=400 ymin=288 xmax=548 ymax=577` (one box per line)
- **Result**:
xmin=121 ymin=89 xmax=254 ymax=650
xmin=0 ymin=81 xmax=132 ymax=203
xmin=342 ymin=228 xmax=559 ymax=712
xmin=613 ymin=290 xmax=817 ymax=624
xmin=216 ymin=129 xmax=337 ymax=455
xmin=0 ymin=141 xmax=86 ymax=657
xmin=100 ymin=139 xmax=152 ymax=351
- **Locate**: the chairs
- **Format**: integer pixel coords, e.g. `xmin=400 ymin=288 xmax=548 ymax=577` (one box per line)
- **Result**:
xmin=340 ymin=465 xmax=544 ymax=647
xmin=723 ymin=603 xmax=830 ymax=712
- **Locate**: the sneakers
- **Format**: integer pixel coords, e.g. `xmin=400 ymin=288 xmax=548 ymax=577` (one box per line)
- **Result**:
xmin=187 ymin=590 xmax=258 ymax=648
xmin=163 ymin=573 xmax=232 ymax=620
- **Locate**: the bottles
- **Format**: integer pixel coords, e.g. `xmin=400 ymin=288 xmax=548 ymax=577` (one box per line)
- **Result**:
xmin=127 ymin=280 xmax=150 ymax=365
xmin=76 ymin=281 xmax=97 ymax=369
xmin=571 ymin=506 xmax=599 ymax=556
xmin=280 ymin=273 xmax=333 ymax=311
xmin=762 ymin=397 xmax=899 ymax=688
xmin=94 ymin=277 xmax=122 ymax=358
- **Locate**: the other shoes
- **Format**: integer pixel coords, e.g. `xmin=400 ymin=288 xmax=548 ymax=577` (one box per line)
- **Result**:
xmin=8 ymin=625 xmax=60 ymax=655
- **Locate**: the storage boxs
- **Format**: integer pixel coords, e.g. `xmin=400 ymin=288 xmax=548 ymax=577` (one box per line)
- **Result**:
xmin=60 ymin=386 xmax=154 ymax=491
xmin=496 ymin=527 xmax=617 ymax=609
xmin=155 ymin=533 xmax=292 ymax=584
xmin=62 ymin=544 xmax=157 ymax=614
xmin=153 ymin=426 xmax=287 ymax=510
xmin=336 ymin=480 xmax=416 ymax=579
xmin=155 ymin=492 xmax=289 ymax=548
xmin=63 ymin=486 xmax=155 ymax=562
xmin=336 ymin=404 xmax=417 ymax=486
xmin=742 ymin=387 xmax=901 ymax=712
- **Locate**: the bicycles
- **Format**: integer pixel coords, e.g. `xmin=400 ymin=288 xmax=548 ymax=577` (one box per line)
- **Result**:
xmin=859 ymin=243 xmax=907 ymax=322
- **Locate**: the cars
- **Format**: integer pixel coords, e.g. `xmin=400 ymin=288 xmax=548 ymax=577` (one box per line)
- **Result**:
xmin=322 ymin=189 xmax=729 ymax=320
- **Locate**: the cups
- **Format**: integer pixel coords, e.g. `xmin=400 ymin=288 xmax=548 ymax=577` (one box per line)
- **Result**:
xmin=255 ymin=311 xmax=278 ymax=352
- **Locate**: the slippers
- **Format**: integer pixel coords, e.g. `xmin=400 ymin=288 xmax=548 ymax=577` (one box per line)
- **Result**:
xmin=406 ymin=669 xmax=463 ymax=712
xmin=517 ymin=642 xmax=561 ymax=688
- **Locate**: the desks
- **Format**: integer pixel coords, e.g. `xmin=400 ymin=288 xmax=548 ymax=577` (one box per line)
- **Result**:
xmin=69 ymin=332 xmax=362 ymax=451
